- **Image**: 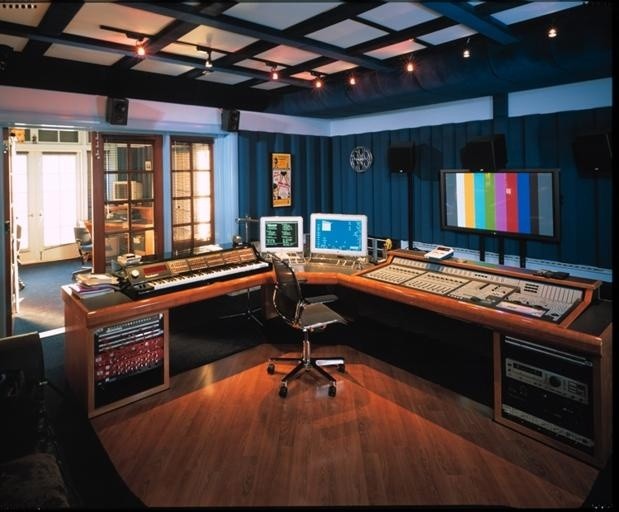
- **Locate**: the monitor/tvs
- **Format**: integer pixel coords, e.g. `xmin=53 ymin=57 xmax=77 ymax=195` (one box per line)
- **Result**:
xmin=113 ymin=181 xmax=143 ymax=204
xmin=260 ymin=216 xmax=303 ymax=259
xmin=310 ymin=213 xmax=367 ymax=258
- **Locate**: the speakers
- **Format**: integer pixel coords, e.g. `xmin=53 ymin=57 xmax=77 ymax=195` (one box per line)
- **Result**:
xmin=572 ymin=128 xmax=612 ymax=178
xmin=388 ymin=141 xmax=416 ymax=173
xmin=466 ymin=133 xmax=507 ymax=171
xmin=221 ymin=109 xmax=239 ymax=131
xmin=106 ymin=97 xmax=128 ymax=125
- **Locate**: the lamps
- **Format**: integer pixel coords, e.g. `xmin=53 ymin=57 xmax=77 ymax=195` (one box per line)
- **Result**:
xmin=205 ymin=52 xmax=213 ymax=68
xmin=407 ymin=53 xmax=413 ymax=72
xmin=548 ymin=23 xmax=556 ymax=38
xmin=136 ymin=37 xmax=146 ymax=56
xmin=349 ymin=70 xmax=356 ymax=85
xmin=463 ymin=37 xmax=470 ymax=58
xmin=272 ymin=64 xmax=278 ymax=80
xmin=315 ymin=76 xmax=321 ymax=88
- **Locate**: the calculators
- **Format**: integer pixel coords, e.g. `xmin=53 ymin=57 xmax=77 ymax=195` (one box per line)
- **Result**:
xmin=424 ymin=245 xmax=454 ymax=261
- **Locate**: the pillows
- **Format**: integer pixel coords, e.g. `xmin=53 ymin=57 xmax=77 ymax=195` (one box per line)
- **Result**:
xmin=74 ymin=227 xmax=91 ymax=245
xmin=0 ymin=453 xmax=72 ymax=512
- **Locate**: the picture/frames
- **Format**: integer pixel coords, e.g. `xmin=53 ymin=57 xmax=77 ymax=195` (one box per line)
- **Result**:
xmin=271 ymin=152 xmax=293 ymax=209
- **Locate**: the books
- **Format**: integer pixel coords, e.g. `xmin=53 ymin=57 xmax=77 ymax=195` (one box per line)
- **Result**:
xmin=68 ymin=272 xmax=121 ymax=300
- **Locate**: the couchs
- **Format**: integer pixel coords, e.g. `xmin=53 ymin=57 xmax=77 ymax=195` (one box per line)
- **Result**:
xmin=0 ymin=332 xmax=91 ymax=512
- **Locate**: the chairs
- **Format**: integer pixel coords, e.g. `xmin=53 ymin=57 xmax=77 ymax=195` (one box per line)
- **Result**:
xmin=268 ymin=252 xmax=353 ymax=397
xmin=76 ymin=219 xmax=92 ymax=265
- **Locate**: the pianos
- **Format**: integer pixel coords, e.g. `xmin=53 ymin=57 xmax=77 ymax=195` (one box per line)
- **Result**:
xmin=112 ymin=245 xmax=273 ymax=301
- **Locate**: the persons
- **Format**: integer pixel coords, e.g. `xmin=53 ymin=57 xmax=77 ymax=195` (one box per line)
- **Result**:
xmin=273 ymin=183 xmax=279 ymax=200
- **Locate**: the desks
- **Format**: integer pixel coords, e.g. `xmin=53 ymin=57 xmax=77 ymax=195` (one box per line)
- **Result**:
xmin=61 ymin=243 xmax=613 ymax=470
xmin=80 ymin=204 xmax=155 ymax=264
